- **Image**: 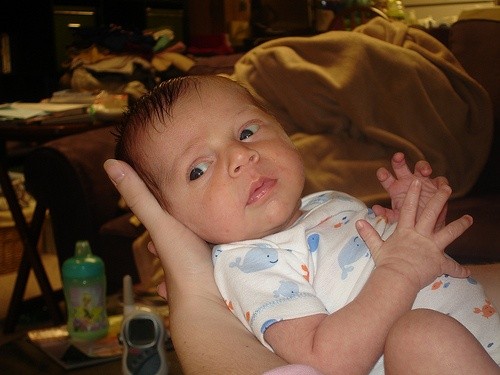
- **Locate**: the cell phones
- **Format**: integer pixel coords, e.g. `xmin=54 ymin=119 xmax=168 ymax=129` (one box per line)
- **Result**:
xmin=121 ymin=274 xmax=167 ymax=375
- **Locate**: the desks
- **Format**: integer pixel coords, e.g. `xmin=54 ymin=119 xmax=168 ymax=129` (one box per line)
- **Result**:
xmin=0 ymin=118 xmax=119 ymax=333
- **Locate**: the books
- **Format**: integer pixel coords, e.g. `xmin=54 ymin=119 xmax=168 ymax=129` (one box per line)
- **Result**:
xmin=0 ymin=99 xmax=93 ymax=127
xmin=26 ymin=306 xmax=174 ymax=372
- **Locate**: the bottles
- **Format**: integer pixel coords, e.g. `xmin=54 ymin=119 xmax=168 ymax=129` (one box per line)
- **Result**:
xmin=63 ymin=242 xmax=109 ymax=340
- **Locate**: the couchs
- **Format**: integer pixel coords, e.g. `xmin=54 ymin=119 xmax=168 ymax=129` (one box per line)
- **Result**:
xmin=22 ymin=17 xmax=500 ymax=264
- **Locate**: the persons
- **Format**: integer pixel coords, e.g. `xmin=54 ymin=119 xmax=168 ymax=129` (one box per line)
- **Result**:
xmin=114 ymin=73 xmax=500 ymax=375
xmin=104 ymin=149 xmax=324 ymax=375
